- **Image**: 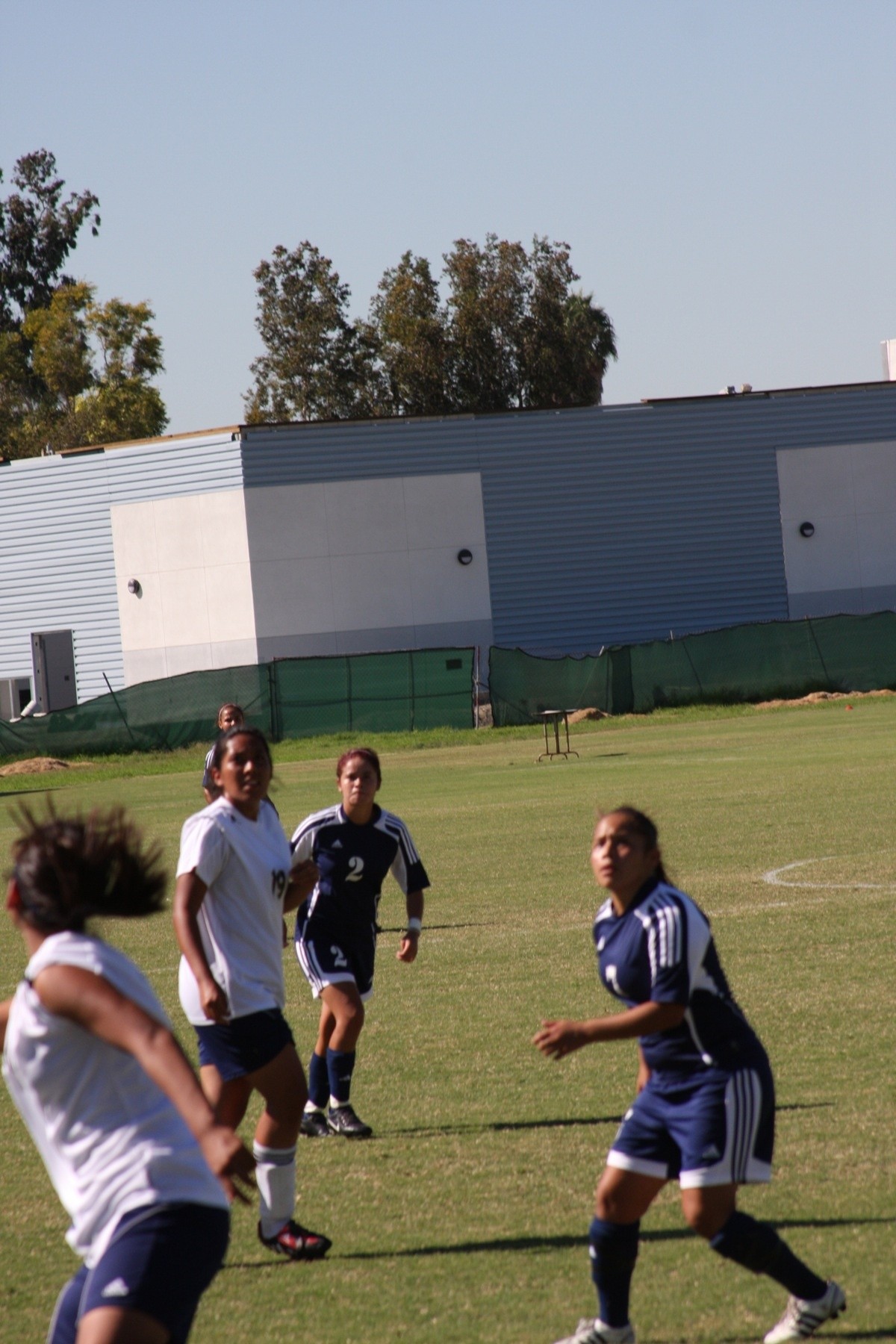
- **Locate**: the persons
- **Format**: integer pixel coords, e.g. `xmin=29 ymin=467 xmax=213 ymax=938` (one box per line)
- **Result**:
xmin=172 ymin=727 xmax=332 ymax=1262
xmin=283 ymin=748 xmax=431 ymax=1141
xmin=529 ymin=805 xmax=848 ymax=1344
xmin=0 ymin=788 xmax=260 ymax=1344
xmin=200 ymin=703 xmax=246 ymax=805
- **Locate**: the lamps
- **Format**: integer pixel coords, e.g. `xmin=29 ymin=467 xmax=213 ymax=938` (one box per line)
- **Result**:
xmin=800 ymin=522 xmax=815 ymax=538
xmin=457 ymin=549 xmax=473 ymax=565
xmin=128 ymin=579 xmax=140 ymax=593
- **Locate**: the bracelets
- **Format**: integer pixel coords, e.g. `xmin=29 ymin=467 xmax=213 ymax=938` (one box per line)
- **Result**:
xmin=408 ymin=917 xmax=421 ymax=934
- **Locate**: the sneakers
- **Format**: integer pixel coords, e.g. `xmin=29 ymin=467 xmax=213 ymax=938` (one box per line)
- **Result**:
xmin=256 ymin=1218 xmax=334 ymax=1261
xmin=298 ymin=1112 xmax=337 ymax=1138
xmin=328 ymin=1104 xmax=374 ymax=1138
xmin=762 ymin=1280 xmax=847 ymax=1344
xmin=555 ymin=1317 xmax=636 ymax=1344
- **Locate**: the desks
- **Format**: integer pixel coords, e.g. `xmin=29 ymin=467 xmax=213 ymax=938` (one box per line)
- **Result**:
xmin=532 ymin=709 xmax=579 ymax=761
